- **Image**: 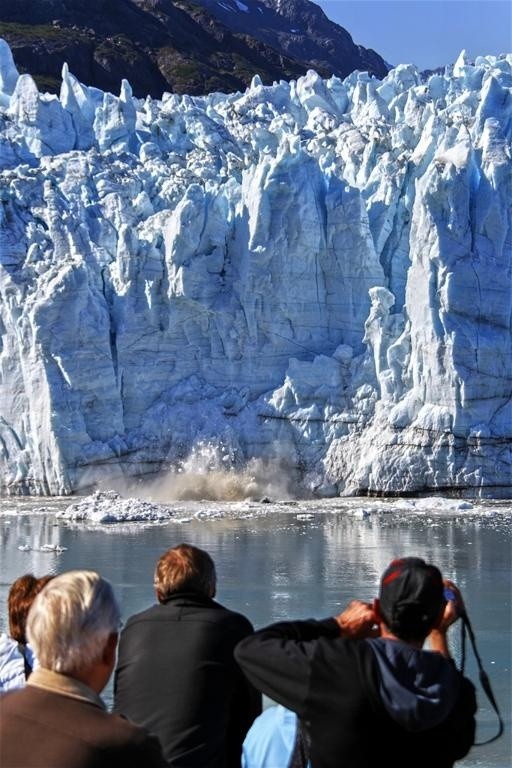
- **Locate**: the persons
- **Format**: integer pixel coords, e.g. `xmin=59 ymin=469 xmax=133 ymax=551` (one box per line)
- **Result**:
xmin=226 ymin=555 xmax=505 ymax=768
xmin=0 ymin=569 xmax=169 ymax=765
xmin=108 ymin=542 xmax=267 ymax=767
xmin=0 ymin=575 xmax=52 ymax=694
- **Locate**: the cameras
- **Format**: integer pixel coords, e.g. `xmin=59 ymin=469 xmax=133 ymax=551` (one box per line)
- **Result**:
xmin=442 ymin=587 xmax=455 ymax=609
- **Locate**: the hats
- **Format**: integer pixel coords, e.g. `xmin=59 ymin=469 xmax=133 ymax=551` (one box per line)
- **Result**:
xmin=379 ymin=558 xmax=446 ymax=631
